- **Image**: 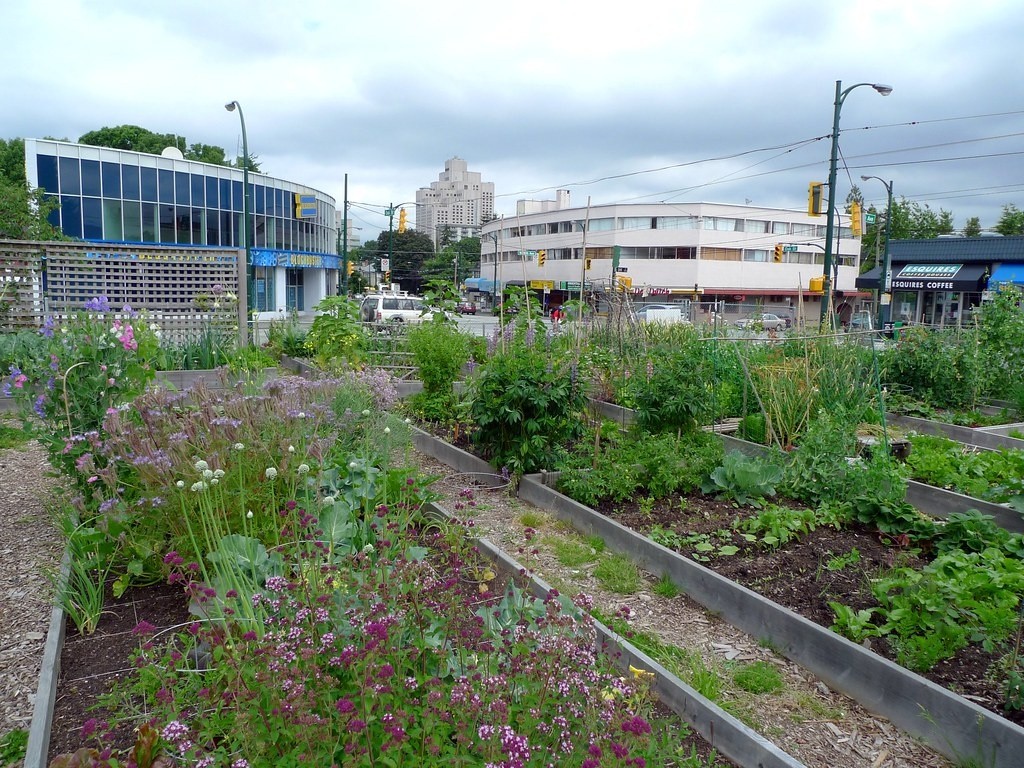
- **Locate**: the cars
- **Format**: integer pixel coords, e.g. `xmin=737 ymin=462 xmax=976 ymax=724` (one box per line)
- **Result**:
xmin=548 ymin=299 xmax=593 ymax=323
xmin=628 ymin=304 xmax=688 ymax=323
xmin=456 ymin=303 xmax=475 ymax=313
xmin=735 ymin=314 xmax=786 ymax=332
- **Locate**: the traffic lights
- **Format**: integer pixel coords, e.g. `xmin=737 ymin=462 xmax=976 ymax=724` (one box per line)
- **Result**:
xmin=538 ymin=251 xmax=546 ymax=266
xmin=694 ymin=283 xmax=698 ymax=291
xmin=385 ymin=271 xmax=389 ymax=283
xmin=348 ymin=261 xmax=354 ymax=275
xmin=399 ymin=209 xmax=407 ymax=234
xmin=585 ymin=258 xmax=592 ymax=270
xmin=774 ymin=244 xmax=782 ymax=263
xmin=848 ymin=202 xmax=862 ymax=236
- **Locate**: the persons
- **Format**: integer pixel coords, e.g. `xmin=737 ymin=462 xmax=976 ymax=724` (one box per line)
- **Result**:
xmin=553 ymin=306 xmax=565 ymax=332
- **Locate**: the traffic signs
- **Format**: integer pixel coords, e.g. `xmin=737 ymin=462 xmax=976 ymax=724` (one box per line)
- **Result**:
xmin=784 ymin=246 xmax=798 ymax=252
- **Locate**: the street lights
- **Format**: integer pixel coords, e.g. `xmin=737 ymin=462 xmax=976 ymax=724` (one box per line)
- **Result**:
xmin=860 ymin=174 xmax=893 ymax=338
xmin=478 ymin=232 xmax=497 ymax=311
xmin=389 ymin=202 xmax=424 ymax=286
xmin=571 ymin=220 xmax=586 ymax=304
xmin=338 ymin=227 xmax=362 ymax=295
xmin=225 ymin=100 xmax=256 ymax=352
xmin=819 ymin=79 xmax=893 ymax=338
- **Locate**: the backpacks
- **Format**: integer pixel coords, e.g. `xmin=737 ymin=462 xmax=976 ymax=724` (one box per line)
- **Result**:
xmin=551 ymin=306 xmax=559 ymax=314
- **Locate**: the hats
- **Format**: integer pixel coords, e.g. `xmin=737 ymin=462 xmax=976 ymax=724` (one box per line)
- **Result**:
xmin=558 ymin=305 xmax=563 ymax=310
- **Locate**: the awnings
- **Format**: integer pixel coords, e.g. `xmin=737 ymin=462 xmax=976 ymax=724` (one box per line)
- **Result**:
xmin=987 ymin=264 xmax=1024 ymax=294
xmin=506 ymin=280 xmax=531 ymax=289
xmin=465 ymin=278 xmax=487 ymax=288
xmin=479 ymin=280 xmax=500 ymax=291
xmin=855 ymin=264 xmax=985 ymax=290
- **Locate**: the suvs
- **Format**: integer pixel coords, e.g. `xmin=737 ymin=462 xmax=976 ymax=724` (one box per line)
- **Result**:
xmin=359 ymin=292 xmax=450 ymax=331
